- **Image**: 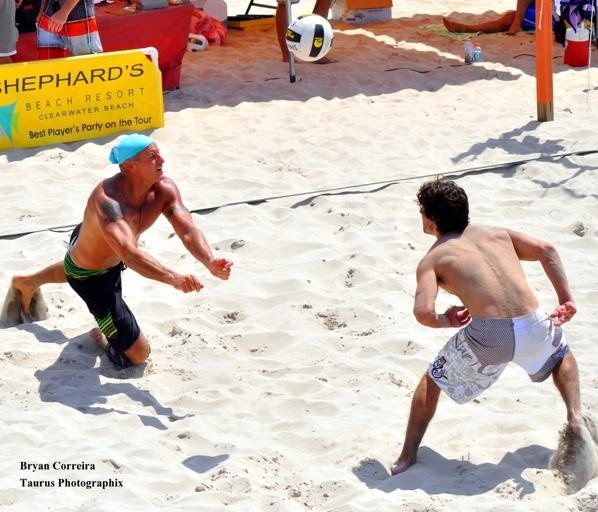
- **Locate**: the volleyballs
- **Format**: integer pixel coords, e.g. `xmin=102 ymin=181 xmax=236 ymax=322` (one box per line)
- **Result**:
xmin=186 ymin=10 xmax=229 ymax=52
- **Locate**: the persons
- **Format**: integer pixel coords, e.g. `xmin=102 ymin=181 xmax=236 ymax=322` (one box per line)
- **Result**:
xmin=274 ymin=1 xmax=340 ymax=64
xmin=15 ymin=1 xmax=41 ymax=34
xmin=505 ymin=0 xmax=531 ymax=36
xmin=12 ymin=133 xmax=234 ymax=365
xmin=34 ymin=1 xmax=104 ymax=60
xmin=389 ymin=178 xmax=585 ymax=475
xmin=0 ymin=1 xmax=24 ymax=64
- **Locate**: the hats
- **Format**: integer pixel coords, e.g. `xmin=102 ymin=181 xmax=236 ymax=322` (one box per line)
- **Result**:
xmin=108 ymin=132 xmax=155 ymax=165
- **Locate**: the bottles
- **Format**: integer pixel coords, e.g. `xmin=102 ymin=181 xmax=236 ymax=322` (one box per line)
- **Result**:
xmin=462 ymin=38 xmax=483 ymax=65
